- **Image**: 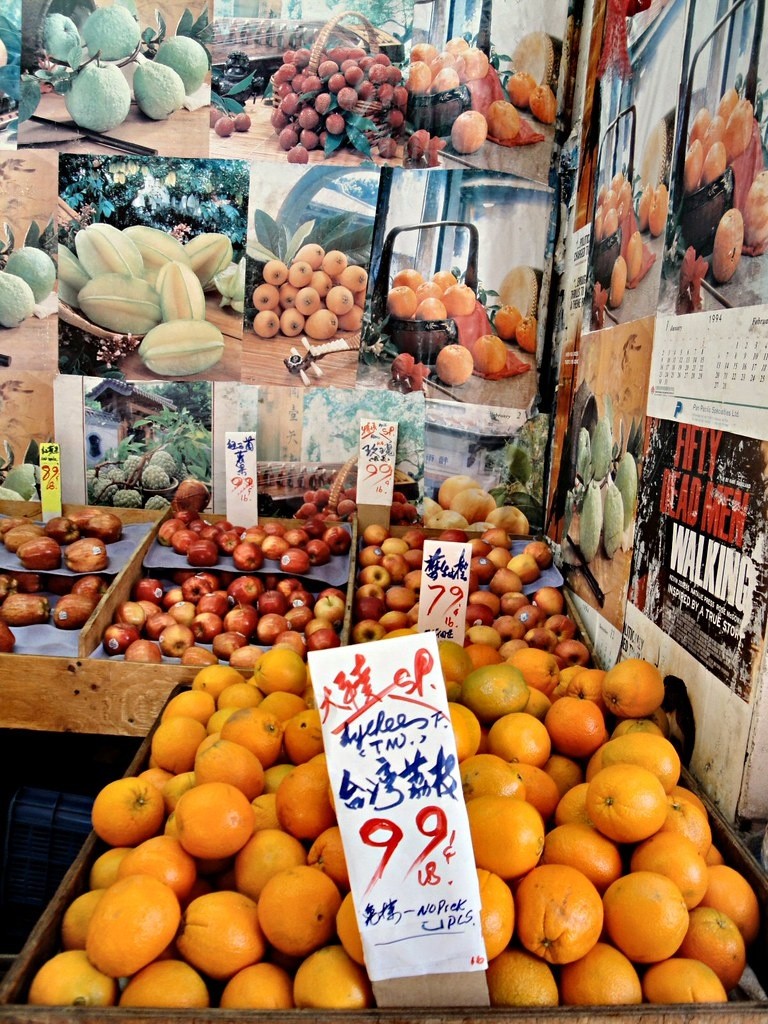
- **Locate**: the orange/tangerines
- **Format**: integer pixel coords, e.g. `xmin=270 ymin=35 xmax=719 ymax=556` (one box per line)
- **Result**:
xmin=592 ymin=89 xmax=768 ymax=308
xmin=292 ymin=486 xmax=421 ymax=527
xmin=26 ymin=625 xmax=760 ymax=1004
xmin=401 ymin=38 xmax=559 ymax=155
xmin=386 ymin=269 xmax=539 ymax=534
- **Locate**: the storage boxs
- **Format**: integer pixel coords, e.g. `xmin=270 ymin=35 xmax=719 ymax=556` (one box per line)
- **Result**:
xmin=85 ymin=512 xmax=358 ymax=736
xmin=0 ymin=660 xmax=767 ymax=1024
xmin=0 ymin=498 xmax=167 ymax=736
xmin=340 ymin=524 xmax=606 ymax=668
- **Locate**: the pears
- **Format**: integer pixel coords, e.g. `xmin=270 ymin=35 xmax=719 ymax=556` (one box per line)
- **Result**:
xmin=577 ymin=416 xmax=638 ymax=564
xmin=40 ymin=5 xmax=210 ymax=133
xmin=0 ymin=247 xmax=56 ymax=329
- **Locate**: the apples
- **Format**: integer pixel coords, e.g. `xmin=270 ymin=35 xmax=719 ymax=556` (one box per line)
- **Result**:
xmin=0 ymin=510 xmax=589 ymax=669
